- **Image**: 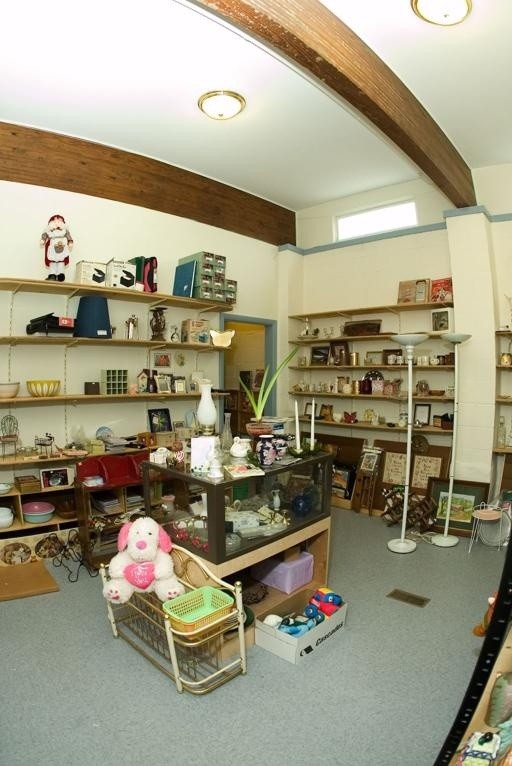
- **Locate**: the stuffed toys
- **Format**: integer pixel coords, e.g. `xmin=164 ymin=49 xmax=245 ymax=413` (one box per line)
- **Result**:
xmin=103 ymin=517 xmax=185 ymax=605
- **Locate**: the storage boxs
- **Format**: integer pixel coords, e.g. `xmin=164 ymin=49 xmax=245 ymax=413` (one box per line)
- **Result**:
xmin=253 ymin=583 xmax=347 ymax=667
xmin=178 ymin=248 xmax=239 ymax=305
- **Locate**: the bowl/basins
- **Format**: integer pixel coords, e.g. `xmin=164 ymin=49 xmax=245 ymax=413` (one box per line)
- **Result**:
xmin=0 ymin=507 xmax=15 ymax=529
xmin=58 ymin=501 xmax=76 ymax=519
xmin=247 ymin=449 xmax=261 ymax=461
xmin=26 ymin=379 xmax=61 ymax=397
xmin=1 ymin=482 xmax=13 ymax=494
xmin=22 ymin=501 xmax=56 ymax=524
xmin=1 ymin=382 xmax=21 ymax=398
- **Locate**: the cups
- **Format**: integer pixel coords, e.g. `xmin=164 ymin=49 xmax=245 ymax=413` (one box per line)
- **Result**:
xmin=386 ymin=354 xmax=430 ymax=367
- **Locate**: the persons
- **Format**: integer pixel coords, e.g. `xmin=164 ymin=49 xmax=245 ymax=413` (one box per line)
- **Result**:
xmin=41 ymin=214 xmax=73 ymax=282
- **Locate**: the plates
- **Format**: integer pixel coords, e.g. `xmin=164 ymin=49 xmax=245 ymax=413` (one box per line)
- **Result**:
xmin=95 ymin=427 xmax=115 ymax=442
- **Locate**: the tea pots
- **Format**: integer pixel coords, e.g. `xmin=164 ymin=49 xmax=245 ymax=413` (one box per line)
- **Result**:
xmin=230 ymin=437 xmax=253 ymax=457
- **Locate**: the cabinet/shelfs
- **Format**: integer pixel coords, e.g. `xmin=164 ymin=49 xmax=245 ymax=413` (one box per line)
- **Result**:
xmin=219 ymin=382 xmax=264 ymax=439
xmin=1 ymin=277 xmax=233 ymax=587
xmin=283 ymin=299 xmax=512 ymax=545
xmin=140 ymin=446 xmax=334 ymax=640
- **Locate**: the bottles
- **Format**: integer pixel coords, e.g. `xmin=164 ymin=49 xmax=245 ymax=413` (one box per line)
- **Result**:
xmin=496 ymin=423 xmax=507 ymax=450
xmin=326 ymin=349 xmax=348 ymax=366
xmin=221 ymin=412 xmax=232 ymax=454
